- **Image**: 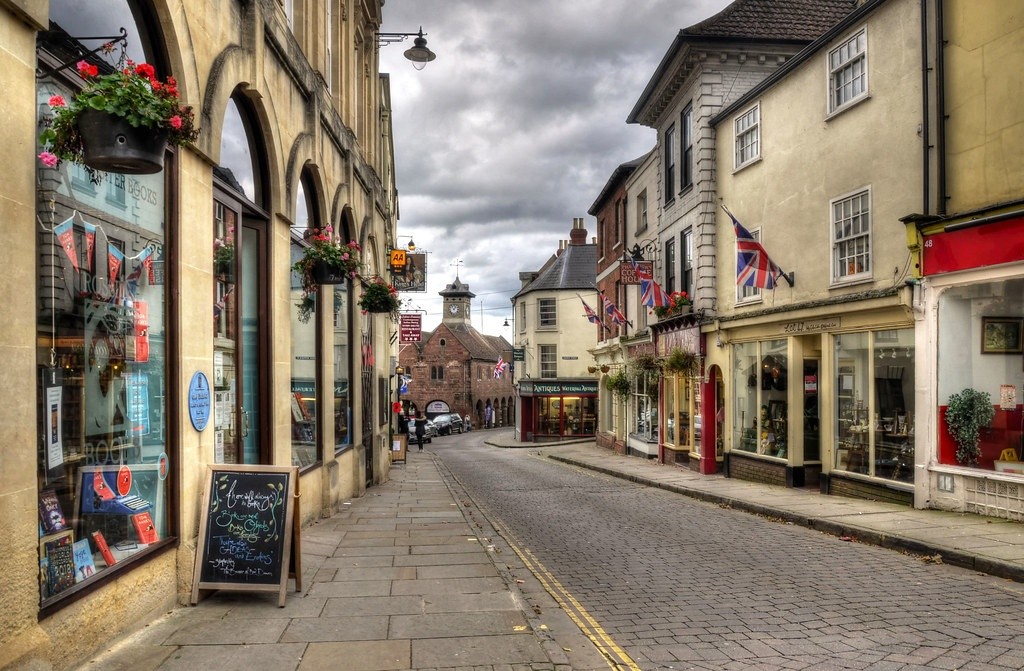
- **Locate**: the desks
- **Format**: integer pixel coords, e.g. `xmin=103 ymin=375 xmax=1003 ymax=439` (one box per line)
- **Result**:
xmin=845 ymin=429 xmax=885 ymax=473
xmin=885 ymin=433 xmax=914 ymax=481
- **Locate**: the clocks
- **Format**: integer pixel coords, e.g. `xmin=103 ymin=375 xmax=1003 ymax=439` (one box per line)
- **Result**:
xmin=449 ymin=304 xmax=459 ymax=316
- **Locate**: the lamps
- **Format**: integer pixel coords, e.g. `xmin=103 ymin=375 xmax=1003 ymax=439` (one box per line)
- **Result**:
xmin=374 ymin=26 xmax=437 ymax=70
xmin=503 ymin=319 xmax=514 ymax=326
xmin=392 ymin=236 xmax=415 ymax=250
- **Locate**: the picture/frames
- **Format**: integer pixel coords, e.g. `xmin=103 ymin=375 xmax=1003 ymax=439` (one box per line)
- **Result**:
xmin=980 ymin=316 xmax=1024 ymax=355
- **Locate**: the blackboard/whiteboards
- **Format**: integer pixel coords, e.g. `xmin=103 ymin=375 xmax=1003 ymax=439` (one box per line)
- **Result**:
xmin=72 ymin=464 xmax=164 ymax=565
xmin=193 ymin=463 xmax=303 ymax=591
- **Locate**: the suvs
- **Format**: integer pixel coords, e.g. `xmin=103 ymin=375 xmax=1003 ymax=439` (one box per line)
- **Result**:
xmin=433 ymin=413 xmax=464 ymax=435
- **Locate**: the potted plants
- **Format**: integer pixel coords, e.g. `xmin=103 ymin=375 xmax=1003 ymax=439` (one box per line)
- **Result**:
xmin=596 ymin=364 xmax=610 ymax=373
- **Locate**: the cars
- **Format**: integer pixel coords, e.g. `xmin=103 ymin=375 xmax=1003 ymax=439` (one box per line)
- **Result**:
xmin=407 ymin=418 xmax=432 ymax=445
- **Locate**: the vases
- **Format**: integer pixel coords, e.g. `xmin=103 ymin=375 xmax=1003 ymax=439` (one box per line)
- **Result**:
xmin=661 ymin=314 xmax=668 ymax=318
xmin=368 ymin=297 xmax=391 ymax=312
xmin=311 ymin=261 xmax=344 ymax=285
xmin=588 ymin=367 xmax=596 ymax=374
xmin=76 ymin=109 xmax=168 ymax=174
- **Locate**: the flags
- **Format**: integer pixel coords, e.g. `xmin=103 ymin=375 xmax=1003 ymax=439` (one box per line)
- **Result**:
xmin=580 ymin=300 xmax=607 ymax=329
xmin=493 ymin=355 xmax=508 ymax=380
xmin=593 ymin=288 xmax=628 ymax=326
xmin=725 ymin=208 xmax=782 ymax=290
xmin=627 ymin=256 xmax=677 ymax=307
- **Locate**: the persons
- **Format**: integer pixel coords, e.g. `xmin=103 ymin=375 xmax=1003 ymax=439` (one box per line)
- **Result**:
xmin=398 ymin=409 xmax=410 ymax=452
xmin=414 ymin=410 xmax=427 ymax=452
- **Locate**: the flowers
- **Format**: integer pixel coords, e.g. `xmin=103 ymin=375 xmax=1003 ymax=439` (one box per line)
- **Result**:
xmin=945 ymin=388 xmax=995 ymax=466
xmin=213 ymin=226 xmax=235 ymax=276
xmin=37 ymin=41 xmax=201 ymax=185
xmin=334 ymin=291 xmax=345 ymax=313
xmin=649 ymin=292 xmax=691 ymax=316
xmin=293 ymin=293 xmax=314 ymax=323
xmin=358 ymin=281 xmax=421 ymax=325
xmin=294 ymin=223 xmax=368 ymax=298
